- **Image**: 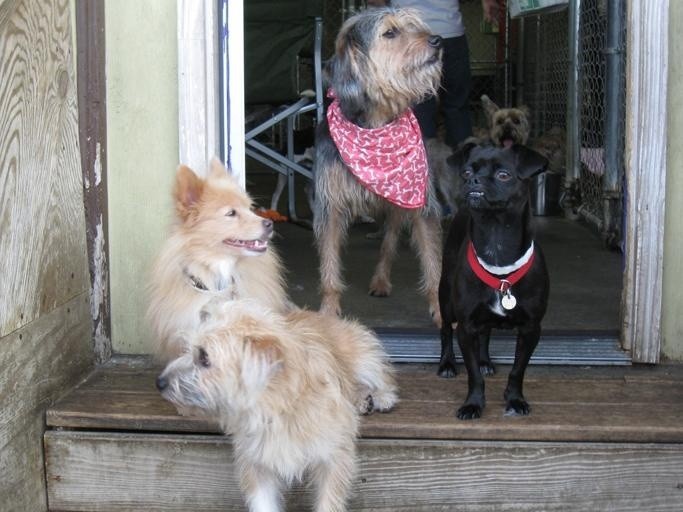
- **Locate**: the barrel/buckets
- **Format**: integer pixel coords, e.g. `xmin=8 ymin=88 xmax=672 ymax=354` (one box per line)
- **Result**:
xmin=529 ymin=169 xmax=562 ymax=217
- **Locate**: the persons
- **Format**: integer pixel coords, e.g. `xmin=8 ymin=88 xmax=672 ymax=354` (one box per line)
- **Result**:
xmin=370 ymin=0 xmax=497 ymax=164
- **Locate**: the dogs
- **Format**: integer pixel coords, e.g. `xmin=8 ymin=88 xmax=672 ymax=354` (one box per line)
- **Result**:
xmin=531 ymin=126 xmax=566 ymax=174
xmin=156 ymin=291 xmax=401 ymax=511
xmin=424 ymin=138 xmax=463 ymax=222
xmin=463 ymin=95 xmax=533 ymax=149
xmin=143 ymin=158 xmax=290 ymax=416
xmin=309 ymin=5 xmax=444 ymax=330
xmin=436 ymin=143 xmax=550 ymax=419
